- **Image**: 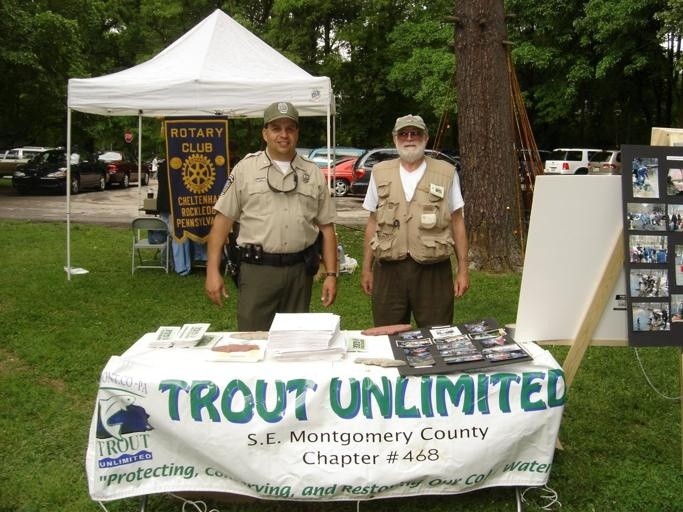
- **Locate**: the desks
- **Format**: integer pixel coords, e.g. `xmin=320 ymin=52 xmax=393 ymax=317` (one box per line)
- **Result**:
xmin=87 ymin=332 xmax=567 ymax=512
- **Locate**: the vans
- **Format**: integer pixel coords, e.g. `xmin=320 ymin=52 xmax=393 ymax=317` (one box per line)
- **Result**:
xmin=307 ymin=148 xmax=366 ymax=168
xmin=587 ymin=150 xmax=621 ymax=175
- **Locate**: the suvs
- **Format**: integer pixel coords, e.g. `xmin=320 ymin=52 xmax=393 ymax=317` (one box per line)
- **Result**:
xmin=349 ymin=147 xmax=462 ymax=198
xmin=544 ymin=147 xmax=601 ymax=174
xmin=1 ymin=147 xmax=47 ymax=162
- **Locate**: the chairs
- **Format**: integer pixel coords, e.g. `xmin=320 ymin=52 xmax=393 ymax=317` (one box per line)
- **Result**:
xmin=131 ymin=216 xmax=172 ymax=273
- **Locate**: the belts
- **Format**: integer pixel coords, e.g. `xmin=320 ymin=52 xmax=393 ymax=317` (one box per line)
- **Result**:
xmin=239 ymin=245 xmax=316 ymax=266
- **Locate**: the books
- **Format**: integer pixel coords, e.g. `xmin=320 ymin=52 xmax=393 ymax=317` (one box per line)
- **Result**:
xmin=268 ymin=312 xmax=346 ymax=362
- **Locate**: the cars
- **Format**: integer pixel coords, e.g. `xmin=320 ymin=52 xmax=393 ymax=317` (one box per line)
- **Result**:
xmin=319 ymin=157 xmax=358 ymax=197
xmin=87 ymin=150 xmax=151 ymax=189
xmin=11 ymin=150 xmax=110 ymax=195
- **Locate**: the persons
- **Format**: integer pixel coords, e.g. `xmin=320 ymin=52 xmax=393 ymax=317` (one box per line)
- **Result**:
xmin=361 ymin=114 xmax=469 ymax=328
xmin=205 ymin=102 xmax=339 ymax=332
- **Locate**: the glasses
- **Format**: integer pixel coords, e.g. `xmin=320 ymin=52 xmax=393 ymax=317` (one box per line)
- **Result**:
xmin=398 ymin=132 xmax=420 ymax=136
xmin=266 ymin=166 xmax=299 ymax=193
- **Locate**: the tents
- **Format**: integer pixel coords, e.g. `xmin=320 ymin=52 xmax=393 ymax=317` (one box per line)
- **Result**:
xmin=62 ymin=10 xmax=334 ymax=285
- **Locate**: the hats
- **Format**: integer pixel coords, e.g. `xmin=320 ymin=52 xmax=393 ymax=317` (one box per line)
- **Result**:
xmin=394 ymin=114 xmax=427 ymax=134
xmin=264 ymin=102 xmax=298 ymax=127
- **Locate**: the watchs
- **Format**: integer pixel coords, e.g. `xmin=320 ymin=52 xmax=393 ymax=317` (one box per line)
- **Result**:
xmin=324 ymin=272 xmax=339 ymax=277
